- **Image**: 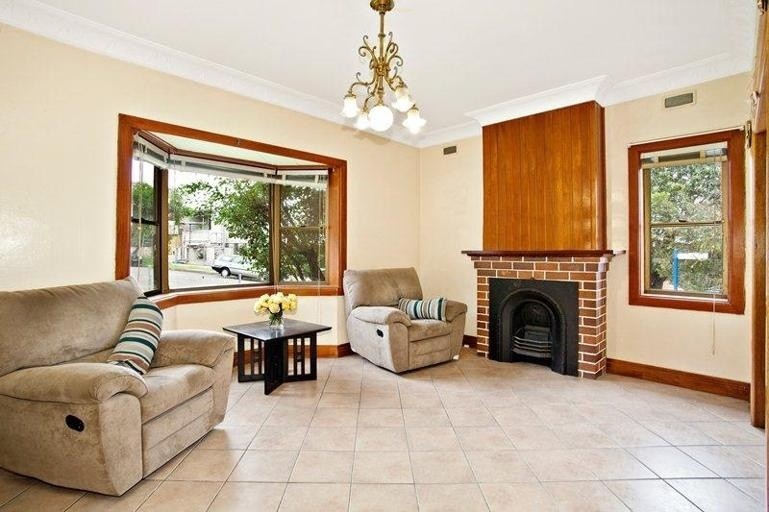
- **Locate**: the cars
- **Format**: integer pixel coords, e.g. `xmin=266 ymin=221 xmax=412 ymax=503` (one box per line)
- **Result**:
xmin=212 ymin=255 xmax=270 ymax=283
xmin=172 ymin=259 xmax=190 ymax=264
xmin=131 ymin=252 xmax=144 ymax=269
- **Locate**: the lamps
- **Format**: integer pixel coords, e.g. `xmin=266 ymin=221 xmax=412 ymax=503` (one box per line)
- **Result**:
xmin=339 ymin=1 xmax=427 ymax=135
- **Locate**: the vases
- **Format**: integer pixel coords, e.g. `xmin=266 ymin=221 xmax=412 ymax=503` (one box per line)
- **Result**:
xmin=268 ymin=311 xmax=284 ymax=330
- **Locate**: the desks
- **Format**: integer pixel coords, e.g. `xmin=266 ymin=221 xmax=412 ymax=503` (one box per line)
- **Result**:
xmin=222 ymin=317 xmax=332 ymax=395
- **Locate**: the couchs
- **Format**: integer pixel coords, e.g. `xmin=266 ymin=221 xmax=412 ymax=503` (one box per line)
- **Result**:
xmin=338 ymin=266 xmax=468 ymax=374
xmin=0 ymin=276 xmax=238 ymax=496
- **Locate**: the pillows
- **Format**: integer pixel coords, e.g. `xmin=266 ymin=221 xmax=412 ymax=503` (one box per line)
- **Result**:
xmin=107 ymin=294 xmax=164 ymax=374
xmin=396 ymin=295 xmax=451 ymax=321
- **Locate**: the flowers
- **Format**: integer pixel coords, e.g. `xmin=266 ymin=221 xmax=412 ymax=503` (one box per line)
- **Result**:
xmin=253 ymin=293 xmax=299 ymax=324
